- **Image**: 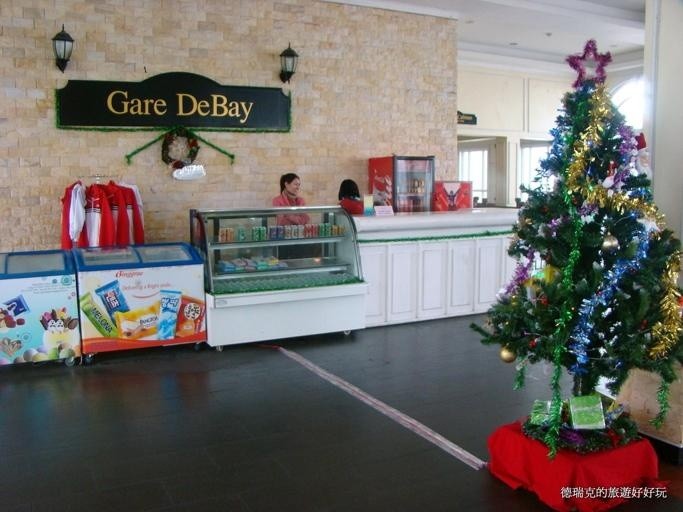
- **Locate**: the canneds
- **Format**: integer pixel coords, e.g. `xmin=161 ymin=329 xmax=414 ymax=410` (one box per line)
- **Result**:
xmin=226 ymin=229 xmax=235 ymax=244
xmin=218 ymin=227 xmax=228 ymax=244
xmin=239 ymin=226 xmax=247 ymax=243
xmin=251 ymin=226 xmax=266 ymax=241
xmin=270 ymin=224 xmax=344 ymax=240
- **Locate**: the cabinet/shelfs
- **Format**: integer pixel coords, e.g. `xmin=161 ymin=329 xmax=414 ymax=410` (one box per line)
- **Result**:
xmin=189 ymin=209 xmax=358 ymax=295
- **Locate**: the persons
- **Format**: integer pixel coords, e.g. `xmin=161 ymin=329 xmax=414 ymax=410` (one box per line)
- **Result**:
xmin=338 ymin=180 xmax=364 ymax=213
xmin=443 ymin=187 xmax=461 ymax=211
xmin=271 ymin=173 xmax=311 ymax=260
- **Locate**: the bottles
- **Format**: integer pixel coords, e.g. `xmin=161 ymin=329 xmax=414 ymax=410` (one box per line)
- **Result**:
xmin=396 ymin=198 xmax=424 ymax=212
xmin=400 ymin=178 xmax=425 ymax=193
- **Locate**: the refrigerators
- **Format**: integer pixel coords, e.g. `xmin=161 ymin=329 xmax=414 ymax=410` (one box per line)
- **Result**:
xmin=0 ymin=249 xmax=81 ymax=367
xmin=368 ymin=155 xmax=435 ymax=212
xmin=71 ymin=241 xmax=207 ymax=362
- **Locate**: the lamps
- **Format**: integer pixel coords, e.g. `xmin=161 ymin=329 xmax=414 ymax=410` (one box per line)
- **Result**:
xmin=276 ymin=40 xmax=300 ymax=87
xmin=49 ymin=20 xmax=76 ymax=74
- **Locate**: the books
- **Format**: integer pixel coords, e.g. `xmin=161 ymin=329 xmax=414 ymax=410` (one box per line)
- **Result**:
xmin=530 ymin=399 xmax=564 ymax=426
xmin=568 ymin=392 xmax=605 ymax=429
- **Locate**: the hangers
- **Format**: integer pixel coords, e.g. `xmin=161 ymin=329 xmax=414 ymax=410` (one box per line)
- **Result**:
xmin=68 ymin=170 xmax=125 ymax=188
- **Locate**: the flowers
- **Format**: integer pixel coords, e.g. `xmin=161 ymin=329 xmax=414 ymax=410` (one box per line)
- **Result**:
xmin=159 ymin=128 xmax=199 ymax=170
